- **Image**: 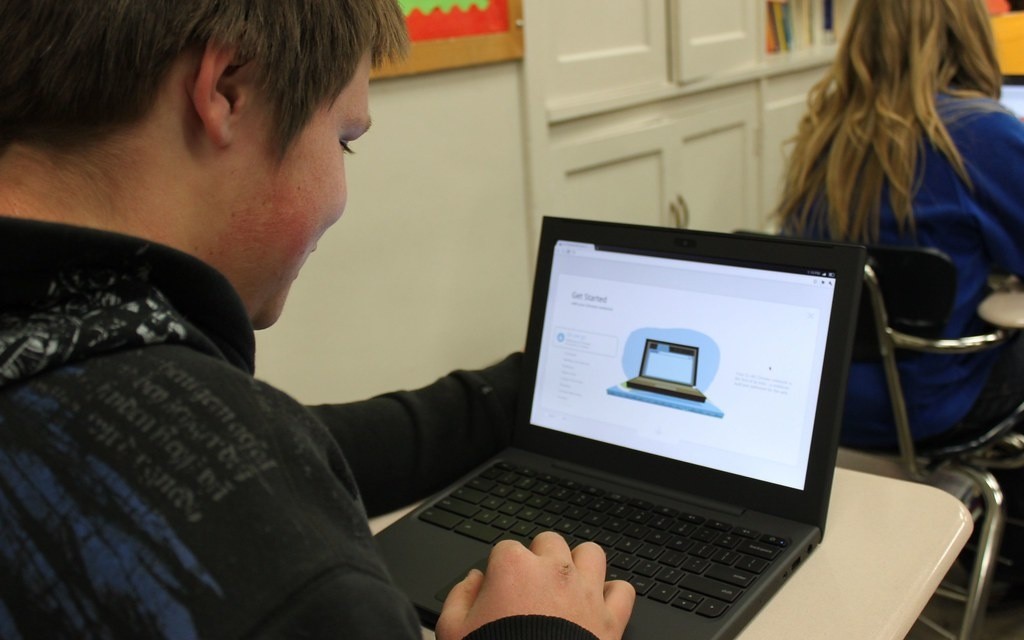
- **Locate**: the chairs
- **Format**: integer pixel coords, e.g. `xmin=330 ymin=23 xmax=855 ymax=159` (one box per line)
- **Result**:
xmin=734 ymin=227 xmax=1024 ymax=640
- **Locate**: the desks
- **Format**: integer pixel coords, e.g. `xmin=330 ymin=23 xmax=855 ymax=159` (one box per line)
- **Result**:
xmin=369 ymin=467 xmax=974 ymax=640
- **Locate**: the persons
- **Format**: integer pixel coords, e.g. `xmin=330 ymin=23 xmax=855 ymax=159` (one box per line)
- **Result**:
xmin=774 ymin=0 xmax=1024 ymax=605
xmin=0 ymin=0 xmax=636 ymax=640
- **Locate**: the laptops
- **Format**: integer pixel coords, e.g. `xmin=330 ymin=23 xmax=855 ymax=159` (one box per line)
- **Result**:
xmin=996 ymin=75 xmax=1024 ymax=123
xmin=626 ymin=339 xmax=707 ymax=404
xmin=373 ymin=214 xmax=867 ymax=640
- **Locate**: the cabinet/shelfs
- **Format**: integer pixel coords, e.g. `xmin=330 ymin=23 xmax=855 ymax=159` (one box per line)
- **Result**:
xmin=527 ymin=0 xmax=858 ymax=237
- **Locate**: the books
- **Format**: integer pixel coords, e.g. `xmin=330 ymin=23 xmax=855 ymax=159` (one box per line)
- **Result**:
xmin=765 ymin=0 xmax=839 ymax=54
